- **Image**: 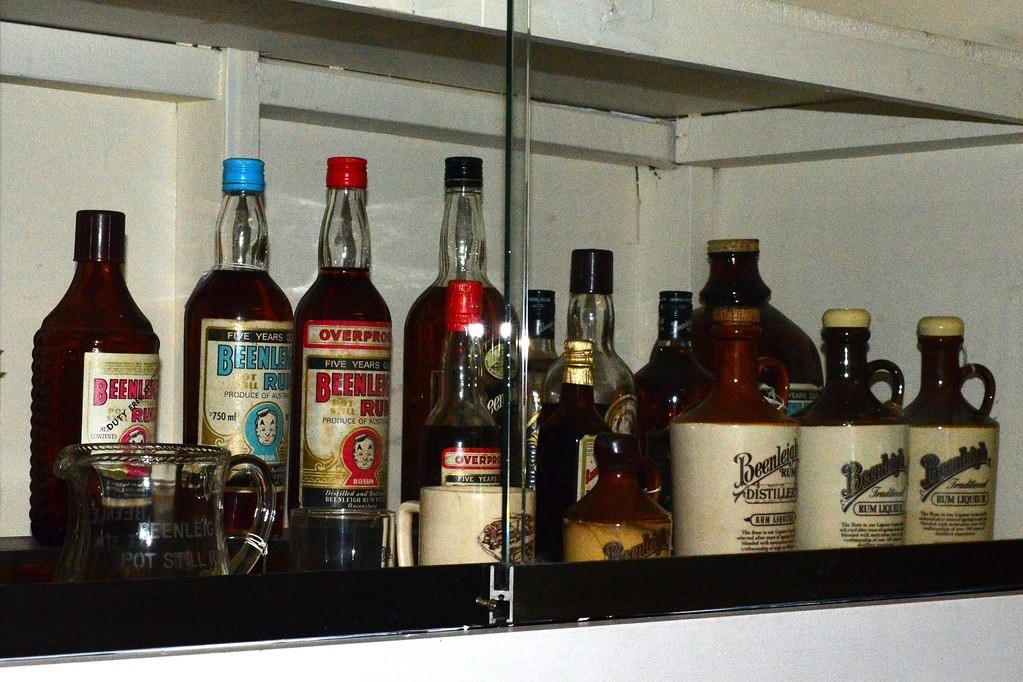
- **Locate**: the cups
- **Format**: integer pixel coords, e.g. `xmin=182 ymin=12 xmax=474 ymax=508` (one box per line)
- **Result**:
xmin=396 ymin=484 xmax=536 ymax=567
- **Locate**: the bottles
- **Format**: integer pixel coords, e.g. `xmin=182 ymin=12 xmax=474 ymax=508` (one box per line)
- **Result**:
xmin=29 ymin=209 xmax=161 ymax=547
xmin=669 ymin=307 xmax=799 ymax=556
xmin=562 ymin=432 xmax=672 ymax=562
xmin=522 ymin=289 xmax=560 ymax=490
xmin=292 ymin=156 xmax=393 ymax=529
xmin=172 ymin=157 xmax=294 ymax=538
xmin=630 ymin=291 xmax=715 ymax=515
xmin=399 ymin=157 xmax=521 ymax=568
xmin=690 ymin=237 xmax=825 ymax=417
xmin=796 ymin=306 xmax=909 ymax=550
xmin=534 ymin=340 xmax=611 ymax=550
xmin=535 ymin=248 xmax=636 ymax=565
xmin=902 ymin=315 xmax=1000 ymax=544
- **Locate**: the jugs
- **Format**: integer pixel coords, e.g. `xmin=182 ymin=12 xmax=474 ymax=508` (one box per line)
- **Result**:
xmin=52 ymin=442 xmax=277 ymax=584
